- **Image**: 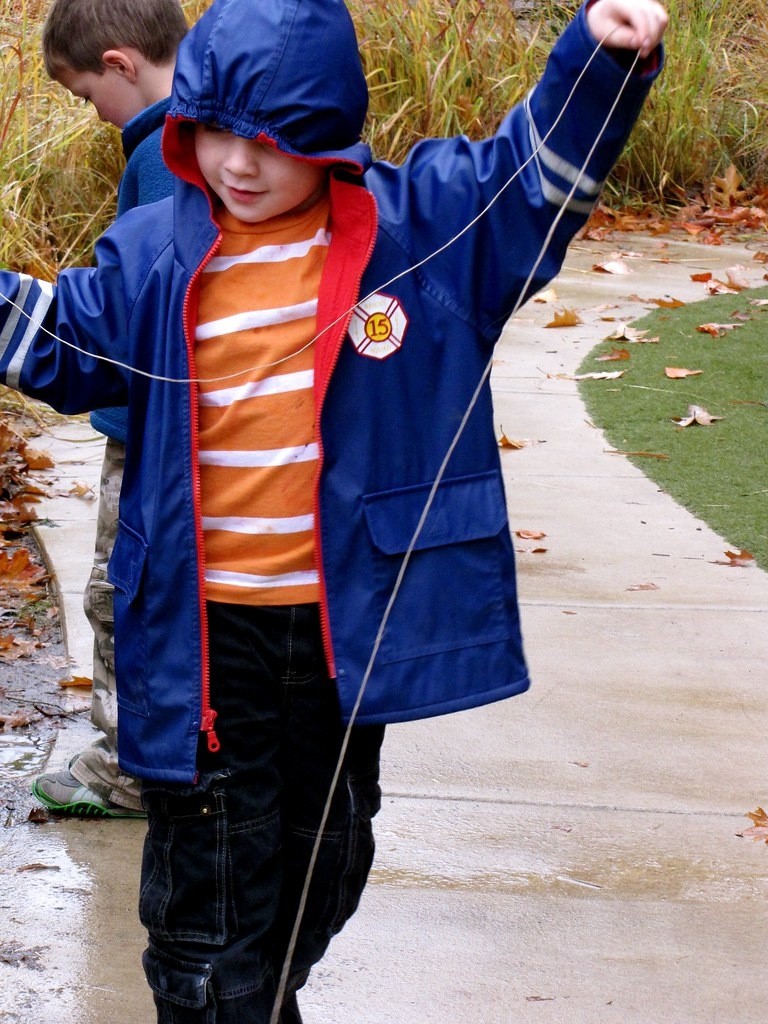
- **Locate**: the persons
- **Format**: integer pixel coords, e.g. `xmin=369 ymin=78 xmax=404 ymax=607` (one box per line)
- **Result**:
xmin=32 ymin=0 xmax=200 ymax=821
xmin=0 ymin=0 xmax=671 ymax=1024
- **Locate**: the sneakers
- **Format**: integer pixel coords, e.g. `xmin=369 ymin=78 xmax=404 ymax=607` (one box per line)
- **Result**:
xmin=32 ymin=771 xmax=147 ymax=820
